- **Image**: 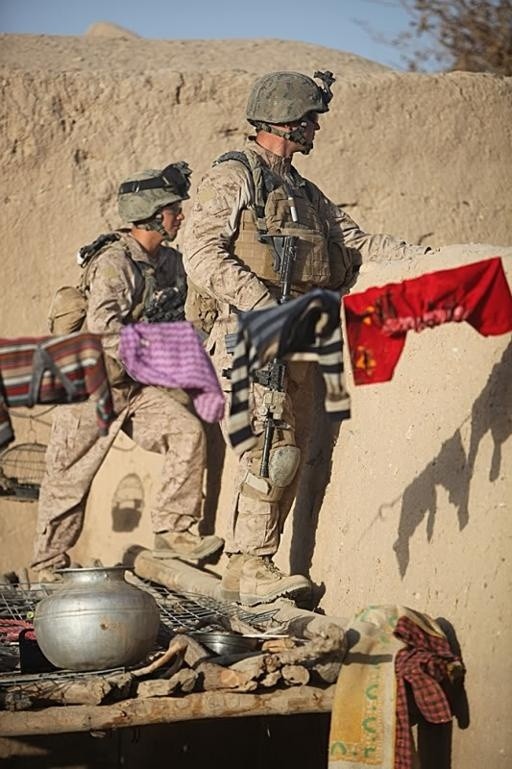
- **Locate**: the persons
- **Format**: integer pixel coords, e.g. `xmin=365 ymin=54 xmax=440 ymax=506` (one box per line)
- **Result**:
xmin=181 ymin=69 xmax=432 ymax=601
xmin=28 ymin=160 xmax=230 ymax=585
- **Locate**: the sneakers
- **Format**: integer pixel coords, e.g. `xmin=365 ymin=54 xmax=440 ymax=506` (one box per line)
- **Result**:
xmin=222 ymin=553 xmax=311 ymax=607
xmin=153 ymin=529 xmax=224 ymax=560
xmin=38 ymin=563 xmax=82 ymax=583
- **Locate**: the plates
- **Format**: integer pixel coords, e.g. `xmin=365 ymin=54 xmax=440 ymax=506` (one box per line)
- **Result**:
xmin=206 ymin=651 xmax=259 ymax=666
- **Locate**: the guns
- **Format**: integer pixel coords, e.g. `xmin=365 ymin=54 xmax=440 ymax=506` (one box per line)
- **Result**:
xmin=222 ymin=235 xmax=299 ymax=477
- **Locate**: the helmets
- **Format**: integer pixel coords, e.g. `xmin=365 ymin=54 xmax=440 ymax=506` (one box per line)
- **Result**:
xmin=119 ymin=163 xmax=190 ymax=194
xmin=118 ymin=168 xmax=190 ymax=223
xmin=245 ymin=70 xmax=328 ymax=123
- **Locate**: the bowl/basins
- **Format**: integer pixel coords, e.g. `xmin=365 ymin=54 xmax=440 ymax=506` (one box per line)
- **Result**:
xmin=193 ymin=632 xmax=260 ymax=653
